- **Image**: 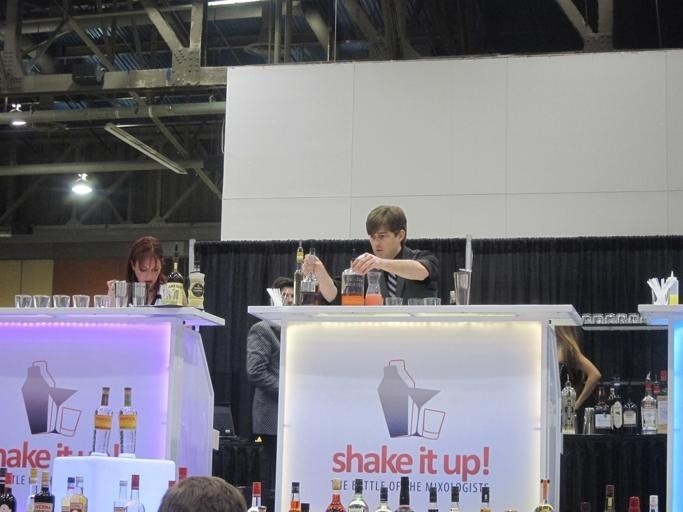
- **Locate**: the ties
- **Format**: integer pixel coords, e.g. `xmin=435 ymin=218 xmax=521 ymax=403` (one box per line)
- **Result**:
xmin=387 ymin=272 xmax=397 ymax=298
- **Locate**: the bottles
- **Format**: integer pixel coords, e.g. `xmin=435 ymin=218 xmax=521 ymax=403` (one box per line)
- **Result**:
xmin=113 ymin=481 xmax=128 ymax=511
xmin=293 ymin=246 xmax=303 ymax=305
xmin=349 ymin=477 xmax=368 ymax=511
xmin=188 ymin=245 xmax=205 ymax=310
xmin=60 ymin=476 xmax=76 ymax=511
xmin=427 ymin=488 xmax=438 ymax=511
xmin=375 ymin=486 xmax=392 ymax=512
xmin=126 ymin=475 xmax=145 ymax=511
xmin=393 ymin=476 xmax=416 ymax=511
xmin=119 ymin=386 xmax=137 ymax=458
xmin=534 ymin=480 xmax=554 ymax=511
xmin=89 ymin=387 xmax=112 ymax=456
xmin=579 ymin=484 xmax=659 ymax=512
xmin=0 ymin=472 xmax=16 ymax=512
xmin=667 ymin=272 xmax=679 ymax=305
xmin=247 ymin=482 xmax=261 ymax=512
xmin=0 ymin=468 xmax=7 ymax=493
xmin=300 ymin=248 xmax=319 ymax=305
xmin=480 ymin=487 xmax=491 ymax=512
xmin=325 ymin=479 xmax=346 ymax=511
xmin=257 ymin=506 xmax=266 ymax=511
xmin=562 ymin=370 xmax=667 ymax=434
xmin=178 ymin=466 xmax=186 ymax=484
xmin=28 ymin=467 xmax=40 ymax=512
xmin=70 ymin=476 xmax=87 ymax=512
xmin=449 ymin=486 xmax=461 ymax=510
xmin=450 ymin=291 xmax=456 ymax=303
xmin=288 ymin=482 xmax=301 ymax=512
xmin=167 ymin=480 xmax=175 ymax=486
xmin=34 ymin=471 xmax=55 ymax=512
xmin=364 ymin=271 xmax=382 ymax=305
xmin=166 ymin=244 xmax=183 ymax=305
xmin=341 ymin=248 xmax=364 ymax=306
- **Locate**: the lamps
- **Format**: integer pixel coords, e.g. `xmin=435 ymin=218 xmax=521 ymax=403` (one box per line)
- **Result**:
xmin=71 ymin=172 xmax=95 ymax=196
xmin=7 ymin=108 xmax=30 ymax=127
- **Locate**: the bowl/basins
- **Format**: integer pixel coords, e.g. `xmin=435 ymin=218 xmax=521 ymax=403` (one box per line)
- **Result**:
xmin=638 ymin=302 xmax=683 ymax=326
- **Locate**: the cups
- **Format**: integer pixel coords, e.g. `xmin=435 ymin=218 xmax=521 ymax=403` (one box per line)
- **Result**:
xmin=406 ymin=299 xmax=423 ymax=306
xmin=13 ymin=294 xmax=109 ymax=307
xmin=424 ymin=297 xmax=441 ymax=305
xmin=453 ymin=271 xmax=470 ymax=306
xmin=579 ymin=313 xmax=642 ymax=325
xmin=114 ymin=281 xmax=127 ymax=308
xmin=385 ymin=296 xmax=403 ymax=306
xmin=131 ymin=283 xmax=146 ymax=306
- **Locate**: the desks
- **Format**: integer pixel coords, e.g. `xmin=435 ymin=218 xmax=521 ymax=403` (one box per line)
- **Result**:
xmin=51 ymin=455 xmax=175 ymax=512
xmin=247 ymin=303 xmax=584 ymax=512
xmin=1 ymin=306 xmax=226 ymax=512
xmin=637 ymin=304 xmax=683 ymax=512
xmin=560 ymin=434 xmax=666 ymax=511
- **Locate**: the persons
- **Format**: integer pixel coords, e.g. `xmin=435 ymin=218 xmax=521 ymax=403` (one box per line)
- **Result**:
xmin=242 ymin=275 xmax=295 ymax=512
xmin=301 ymin=204 xmax=440 ymax=307
xmin=553 ymin=327 xmax=602 ymax=414
xmin=123 ymin=233 xmax=169 ymax=308
xmin=159 ymin=475 xmax=252 ymax=512
